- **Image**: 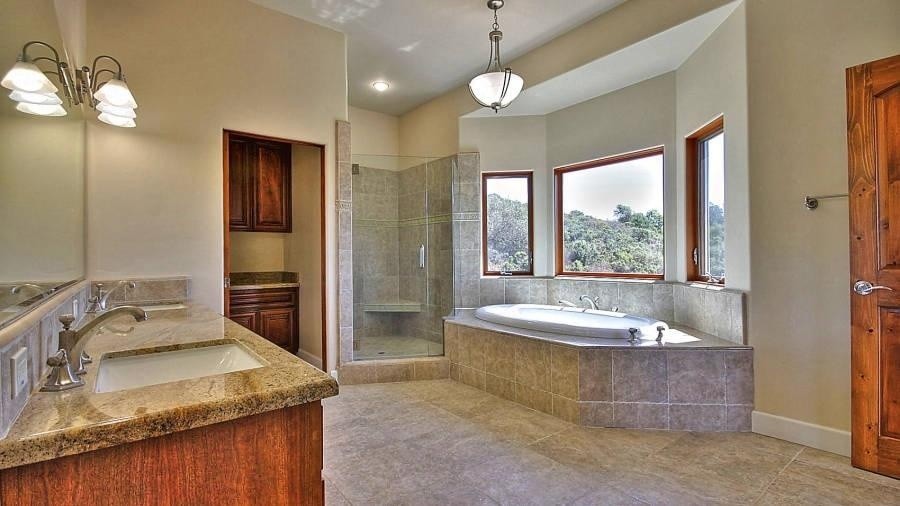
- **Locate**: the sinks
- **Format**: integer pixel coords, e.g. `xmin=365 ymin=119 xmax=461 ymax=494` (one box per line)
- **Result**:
xmin=112 ymin=301 xmax=192 ymax=312
xmin=93 ymin=339 xmax=271 ymax=395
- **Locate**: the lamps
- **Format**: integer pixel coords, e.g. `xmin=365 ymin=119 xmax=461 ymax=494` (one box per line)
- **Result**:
xmin=81 ymin=51 xmax=139 ymax=132
xmin=467 ymin=1 xmax=525 ymax=115
xmin=2 ymin=34 xmax=79 ymax=121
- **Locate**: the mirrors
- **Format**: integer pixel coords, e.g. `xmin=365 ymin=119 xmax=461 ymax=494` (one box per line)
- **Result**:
xmin=0 ymin=0 xmax=89 ymax=345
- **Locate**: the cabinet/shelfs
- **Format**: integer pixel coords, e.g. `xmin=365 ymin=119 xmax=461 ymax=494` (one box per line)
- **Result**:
xmin=232 ymin=306 xmax=296 ymax=356
xmin=225 ymin=135 xmax=294 ymax=232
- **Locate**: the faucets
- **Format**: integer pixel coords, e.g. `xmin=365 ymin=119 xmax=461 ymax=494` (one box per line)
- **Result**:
xmin=59 ymin=305 xmax=146 ymax=375
xmin=96 ymin=280 xmax=135 ymax=309
xmin=580 ymin=294 xmax=599 ymax=310
xmin=12 ymin=284 xmax=44 ymax=294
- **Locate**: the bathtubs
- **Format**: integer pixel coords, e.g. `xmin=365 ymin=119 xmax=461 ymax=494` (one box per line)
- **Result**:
xmin=475 ymin=303 xmax=669 ymax=339
